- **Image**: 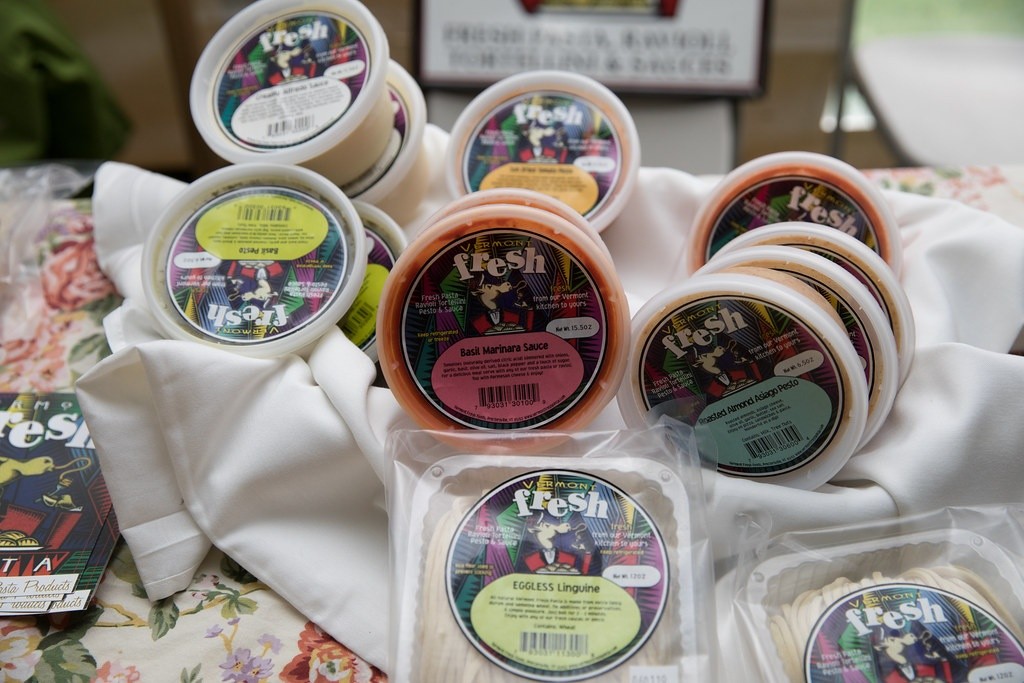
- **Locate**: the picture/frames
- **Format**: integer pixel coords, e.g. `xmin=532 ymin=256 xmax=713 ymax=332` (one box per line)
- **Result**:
xmin=408 ymin=0 xmax=771 ymax=100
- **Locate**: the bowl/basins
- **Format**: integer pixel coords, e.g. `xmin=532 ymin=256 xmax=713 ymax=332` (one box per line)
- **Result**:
xmin=140 ymin=0 xmax=916 ymax=492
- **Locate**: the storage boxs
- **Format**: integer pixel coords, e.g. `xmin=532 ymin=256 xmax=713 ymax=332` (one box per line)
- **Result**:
xmin=396 ymin=455 xmax=699 ymax=683
xmin=745 ymin=527 xmax=1024 ymax=683
